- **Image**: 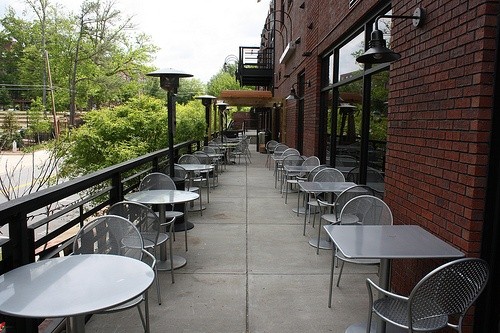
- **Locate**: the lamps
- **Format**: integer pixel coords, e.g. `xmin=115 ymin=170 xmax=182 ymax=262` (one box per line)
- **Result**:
xmin=286 ymin=79 xmax=310 ymax=99
xmin=356 ymin=8 xmax=425 ymax=64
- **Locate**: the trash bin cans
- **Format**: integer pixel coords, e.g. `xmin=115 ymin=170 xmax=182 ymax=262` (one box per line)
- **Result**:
xmin=258 ymin=132 xmax=266 ymax=154
xmin=223 ymin=131 xmax=243 ymax=138
xmin=265 ymin=130 xmax=272 ymax=151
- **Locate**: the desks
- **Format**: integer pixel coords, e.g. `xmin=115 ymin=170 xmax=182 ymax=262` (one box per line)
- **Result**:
xmin=175 ymin=164 xmax=214 ymax=211
xmin=0 ymin=253 xmax=155 ymax=333
xmin=323 ymin=223 xmax=465 ymax=333
xmin=208 ymin=143 xmax=240 ymax=158
xmin=336 ymin=166 xmax=384 ymax=185
xmin=298 ymin=181 xmax=367 ymax=251
xmin=272 ymin=155 xmax=308 ymax=193
xmin=326 ymin=158 xmax=359 ymax=163
xmin=367 ymin=183 xmax=384 ymax=196
xmin=195 ymin=153 xmax=225 ymax=188
xmin=210 ymin=146 xmax=236 ymax=165
xmin=124 ymin=190 xmax=200 ymax=270
xmin=284 ymin=165 xmax=331 ymax=214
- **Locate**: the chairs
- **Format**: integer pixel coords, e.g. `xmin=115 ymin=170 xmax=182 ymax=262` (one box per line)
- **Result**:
xmin=345 ymin=167 xmax=384 ymax=185
xmin=66 ymin=135 xmax=489 ymax=333
